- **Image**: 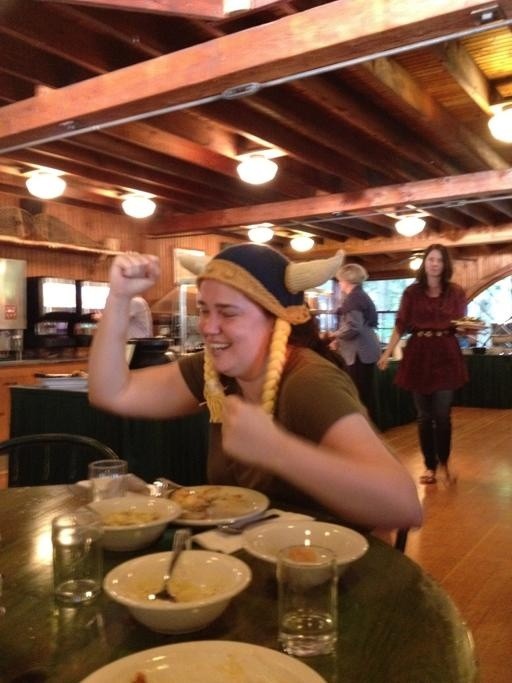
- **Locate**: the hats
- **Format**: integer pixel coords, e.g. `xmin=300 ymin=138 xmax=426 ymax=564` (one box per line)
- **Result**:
xmin=178 ymin=243 xmax=345 ymax=325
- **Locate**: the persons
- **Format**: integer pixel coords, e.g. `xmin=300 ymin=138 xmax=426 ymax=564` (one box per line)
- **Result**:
xmin=376 ymin=245 xmax=481 ymax=488
xmin=89 ymin=243 xmax=425 ymax=546
xmin=90 ymin=295 xmax=153 ymax=339
xmin=320 ymin=264 xmax=381 ymax=425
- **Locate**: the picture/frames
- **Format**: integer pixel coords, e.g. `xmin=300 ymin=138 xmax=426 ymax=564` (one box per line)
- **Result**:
xmin=170 ymin=245 xmax=207 ymax=287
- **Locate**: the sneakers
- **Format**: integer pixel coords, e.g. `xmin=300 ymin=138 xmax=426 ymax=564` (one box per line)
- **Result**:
xmin=420 ymin=472 xmax=436 ymax=483
xmin=444 ymin=473 xmax=457 ymax=484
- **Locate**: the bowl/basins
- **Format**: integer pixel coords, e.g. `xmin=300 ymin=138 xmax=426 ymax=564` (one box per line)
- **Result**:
xmin=108 ymin=549 xmax=252 ymax=630
xmin=471 ymin=347 xmax=486 ymax=354
xmin=169 ymin=487 xmax=270 ymax=524
xmin=77 ymin=495 xmax=182 ymax=553
xmin=243 ymin=519 xmax=370 ymax=588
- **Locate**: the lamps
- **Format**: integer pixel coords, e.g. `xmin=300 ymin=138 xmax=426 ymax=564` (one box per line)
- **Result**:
xmin=408 ymin=250 xmax=425 ymax=271
xmin=395 ymin=208 xmax=426 ymax=237
xmin=289 ymin=232 xmax=315 ymax=252
xmin=234 ymin=144 xmax=278 ymax=186
xmin=246 ymin=222 xmax=273 ymax=243
xmin=485 ymin=95 xmax=512 ymax=143
xmin=116 ymin=186 xmax=159 ymax=219
xmin=23 ymin=167 xmax=65 ymax=200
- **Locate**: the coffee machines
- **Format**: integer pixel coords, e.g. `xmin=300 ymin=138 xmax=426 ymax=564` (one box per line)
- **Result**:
xmin=72 ymin=278 xmax=113 ymax=346
xmin=23 ymin=274 xmax=79 ymax=348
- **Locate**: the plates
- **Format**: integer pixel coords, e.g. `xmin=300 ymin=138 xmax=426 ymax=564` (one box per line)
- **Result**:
xmin=451 ymin=321 xmax=489 ymax=330
xmin=79 ymin=640 xmax=327 ymax=683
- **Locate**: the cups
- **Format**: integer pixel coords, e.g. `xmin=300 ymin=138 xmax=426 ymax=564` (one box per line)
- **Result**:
xmin=102 ymin=236 xmax=121 ymax=251
xmin=51 ymin=512 xmax=104 ymax=605
xmin=88 ymin=459 xmax=126 ymax=501
xmin=276 ymin=545 xmax=338 ymax=658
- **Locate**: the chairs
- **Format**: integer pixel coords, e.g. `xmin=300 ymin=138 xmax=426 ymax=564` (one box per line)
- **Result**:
xmin=0 ymin=433 xmax=124 ymax=488
xmin=300 ymin=492 xmax=409 ymax=554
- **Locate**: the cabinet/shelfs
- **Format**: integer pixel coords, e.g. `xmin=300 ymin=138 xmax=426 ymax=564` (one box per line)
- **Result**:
xmin=0 ymin=361 xmax=91 ymax=445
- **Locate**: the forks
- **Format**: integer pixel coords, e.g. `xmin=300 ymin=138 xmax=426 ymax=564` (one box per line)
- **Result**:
xmin=219 ymin=513 xmax=281 ymax=535
